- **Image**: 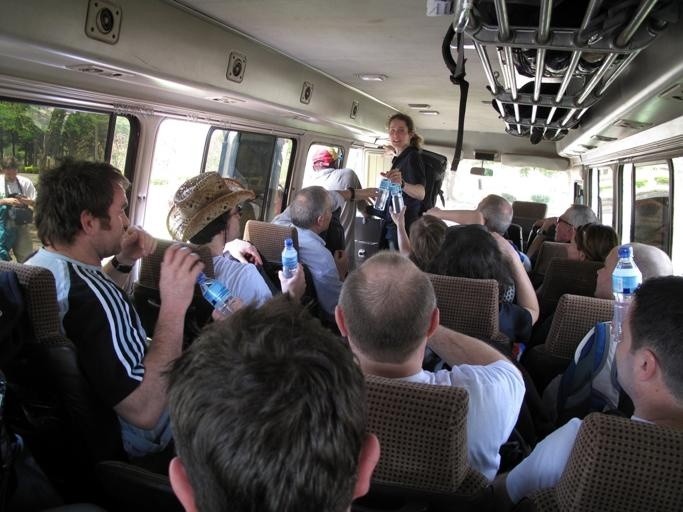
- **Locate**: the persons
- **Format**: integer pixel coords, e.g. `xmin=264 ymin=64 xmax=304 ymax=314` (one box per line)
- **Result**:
xmin=305 ymin=146 xmax=369 ymax=284
xmin=164 ymin=170 xmax=309 ymax=309
xmin=388 ymin=195 xmax=673 ymax=352
xmin=270 ymin=185 xmax=381 ymax=317
xmin=20 ymin=155 xmax=205 ymax=456
xmin=486 ymin=274 xmax=682 ymax=512
xmin=377 ymin=110 xmax=428 ymax=252
xmin=0 ymin=155 xmax=37 ymax=263
xmin=167 ymin=292 xmax=381 ymax=512
xmin=333 ymin=248 xmax=526 ymax=483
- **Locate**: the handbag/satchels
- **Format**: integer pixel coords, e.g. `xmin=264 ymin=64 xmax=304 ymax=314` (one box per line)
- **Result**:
xmin=419 ymin=147 xmax=447 ymax=211
xmin=4 ymin=193 xmax=34 ymax=224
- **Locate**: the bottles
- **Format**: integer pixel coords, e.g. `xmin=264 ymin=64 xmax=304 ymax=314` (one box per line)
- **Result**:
xmin=611 ymin=245 xmax=642 ymax=342
xmin=281 ymin=238 xmax=297 ymax=279
xmin=389 ymin=178 xmax=405 ymax=214
xmin=197 ymin=272 xmax=241 ymax=317
xmin=373 ymin=171 xmax=391 ymax=211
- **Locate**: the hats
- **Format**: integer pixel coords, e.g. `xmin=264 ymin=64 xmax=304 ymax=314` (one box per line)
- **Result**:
xmin=313 ymin=148 xmax=339 ymax=167
xmin=166 ymin=170 xmax=257 ymax=243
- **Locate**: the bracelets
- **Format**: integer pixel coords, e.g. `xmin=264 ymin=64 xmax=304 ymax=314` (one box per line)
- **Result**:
xmin=402 ymin=179 xmax=405 ymax=189
xmin=347 ymin=186 xmax=356 ymax=202
xmin=110 ymin=256 xmax=135 ymax=274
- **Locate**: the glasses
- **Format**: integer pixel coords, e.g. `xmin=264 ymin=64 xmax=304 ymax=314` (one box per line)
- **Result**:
xmin=229 ymin=207 xmax=241 ymax=217
xmin=556 ymin=217 xmax=571 ymax=226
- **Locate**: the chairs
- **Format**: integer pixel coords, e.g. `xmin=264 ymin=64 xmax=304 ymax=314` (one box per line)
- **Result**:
xmin=132 ymin=236 xmax=214 ymax=345
xmin=503 ymin=196 xmax=557 ymax=253
xmin=351 ymin=215 xmax=385 ymax=270
xmin=514 ymin=410 xmax=682 ymax=512
xmin=0 ymin=254 xmax=86 ymax=389
xmin=362 ymin=376 xmax=493 ymax=496
xmin=242 ymin=218 xmax=318 ymax=306
xmin=519 ymin=291 xmax=618 ymax=390
xmin=426 ymin=271 xmax=511 ymax=359
xmin=535 ymin=257 xmax=604 ymax=323
